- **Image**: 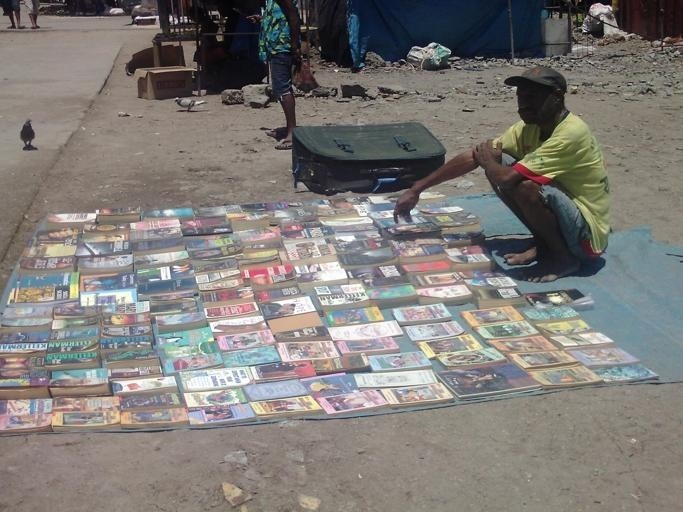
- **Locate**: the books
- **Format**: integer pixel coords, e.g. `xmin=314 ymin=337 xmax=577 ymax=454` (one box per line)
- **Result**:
xmin=0 ymin=189 xmax=659 ymax=434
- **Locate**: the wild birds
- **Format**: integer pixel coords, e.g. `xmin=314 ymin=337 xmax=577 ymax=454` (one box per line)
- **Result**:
xmin=19 ymin=117 xmax=35 ymax=149
xmin=173 ymin=97 xmax=209 ymax=112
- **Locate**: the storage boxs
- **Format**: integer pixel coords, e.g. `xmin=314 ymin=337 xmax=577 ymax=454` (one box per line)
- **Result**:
xmin=124 ymin=44 xmax=198 ymax=101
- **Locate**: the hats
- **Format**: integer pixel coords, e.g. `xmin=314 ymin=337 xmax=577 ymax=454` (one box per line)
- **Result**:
xmin=504 ymin=67 xmax=568 ymax=97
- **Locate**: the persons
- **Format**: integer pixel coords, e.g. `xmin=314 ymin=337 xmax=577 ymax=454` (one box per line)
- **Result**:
xmin=393 ymin=68 xmax=612 ymax=283
xmin=245 ymin=0 xmax=302 ymax=150
xmin=0 ymin=0 xmax=42 ymax=29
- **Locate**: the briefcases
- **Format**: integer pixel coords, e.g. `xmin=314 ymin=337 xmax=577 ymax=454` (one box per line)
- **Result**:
xmin=292 ymin=123 xmax=445 ymax=195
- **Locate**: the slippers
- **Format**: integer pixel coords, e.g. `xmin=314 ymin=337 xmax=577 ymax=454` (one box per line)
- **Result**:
xmin=275 ymin=140 xmax=291 ymax=150
